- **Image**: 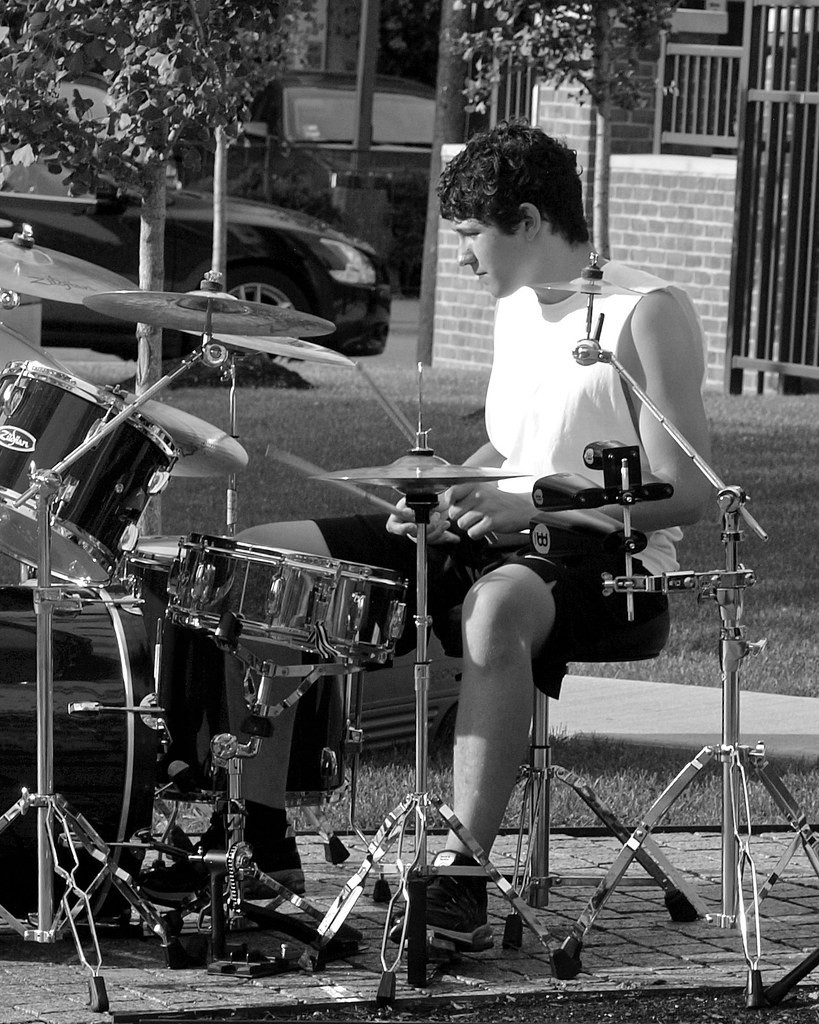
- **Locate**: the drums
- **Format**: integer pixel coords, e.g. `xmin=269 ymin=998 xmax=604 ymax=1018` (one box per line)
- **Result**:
xmin=119 ymin=534 xmax=353 ymax=806
xmin=0 ymin=321 xmax=77 ymax=376
xmin=0 ymin=576 xmax=159 ymax=928
xmin=165 ymin=532 xmax=411 ymax=667
xmin=0 ymin=358 xmax=183 ymax=587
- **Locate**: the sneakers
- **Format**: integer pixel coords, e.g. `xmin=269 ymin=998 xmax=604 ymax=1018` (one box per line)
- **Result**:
xmin=134 ymin=822 xmax=306 ymax=905
xmin=387 ymin=849 xmax=495 ymax=952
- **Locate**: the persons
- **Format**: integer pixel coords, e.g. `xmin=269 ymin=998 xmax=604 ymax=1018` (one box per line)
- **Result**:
xmin=139 ymin=118 xmax=711 ymax=952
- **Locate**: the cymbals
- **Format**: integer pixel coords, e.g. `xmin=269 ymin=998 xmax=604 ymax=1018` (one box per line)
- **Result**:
xmin=181 ymin=329 xmax=355 ymax=367
xmin=309 ymin=455 xmax=534 ymax=494
xmin=0 ymin=237 xmax=140 ymax=307
xmin=39 ymin=346 xmax=137 ymax=385
xmin=84 ymin=289 xmax=337 ymax=338
xmin=524 ymin=277 xmax=652 ymax=296
xmin=121 ymin=393 xmax=248 ymax=479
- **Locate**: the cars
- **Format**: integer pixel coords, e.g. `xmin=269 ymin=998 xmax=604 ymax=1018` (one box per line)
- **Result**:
xmin=0 ymin=71 xmax=438 ymax=355
